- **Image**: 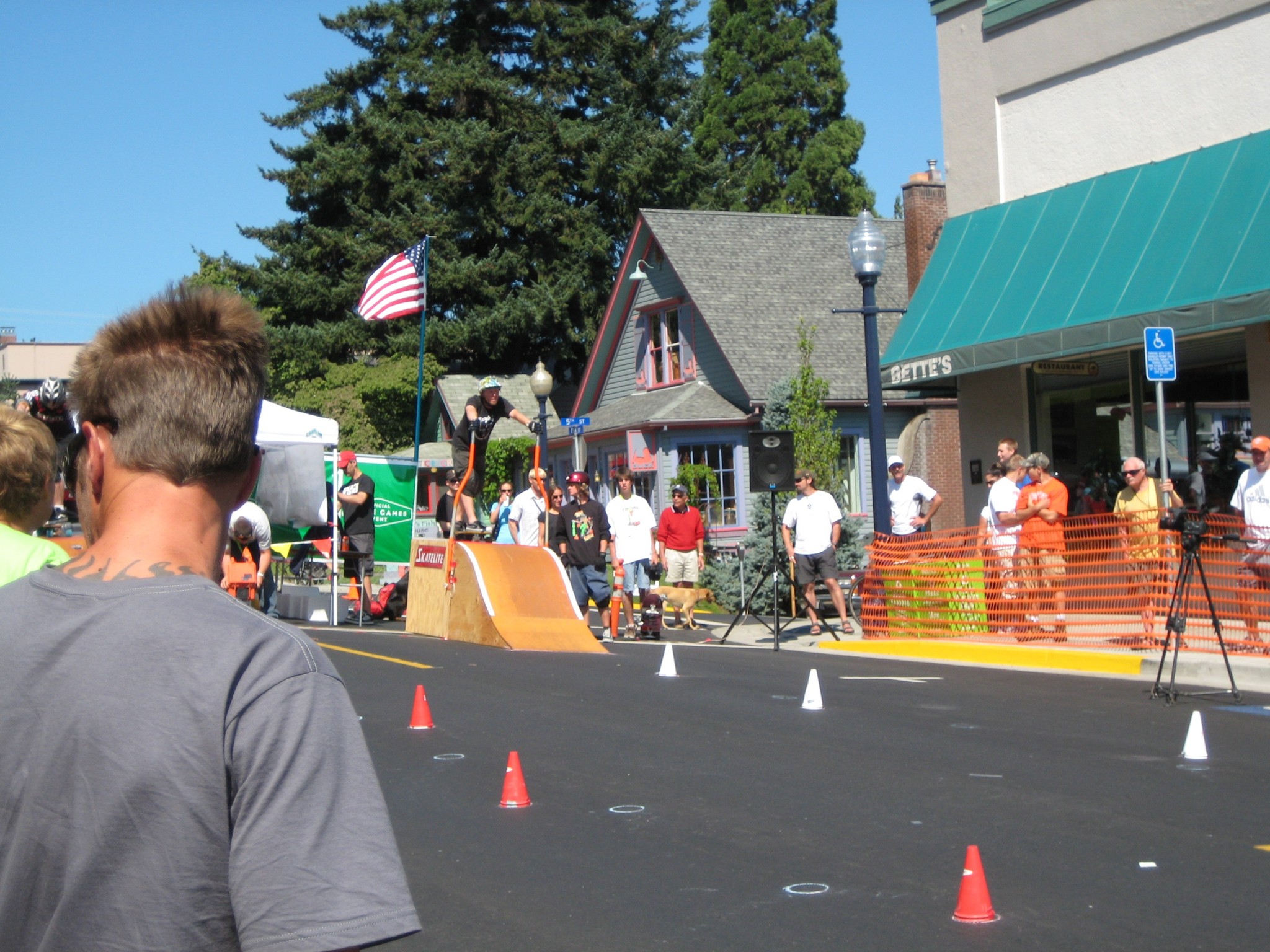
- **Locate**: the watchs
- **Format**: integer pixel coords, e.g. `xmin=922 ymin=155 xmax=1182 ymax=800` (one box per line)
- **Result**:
xmin=832 ymin=542 xmax=839 ymax=547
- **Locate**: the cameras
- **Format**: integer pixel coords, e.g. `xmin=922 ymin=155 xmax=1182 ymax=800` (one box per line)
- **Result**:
xmin=1159 ymin=514 xmax=1206 ymax=551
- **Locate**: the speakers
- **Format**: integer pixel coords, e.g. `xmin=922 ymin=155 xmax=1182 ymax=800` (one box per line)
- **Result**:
xmin=747 ymin=430 xmax=796 ymax=492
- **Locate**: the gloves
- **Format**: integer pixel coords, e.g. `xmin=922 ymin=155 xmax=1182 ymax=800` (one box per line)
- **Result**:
xmin=529 ymin=421 xmax=543 ymax=434
xmin=468 ymin=419 xmax=481 ymax=431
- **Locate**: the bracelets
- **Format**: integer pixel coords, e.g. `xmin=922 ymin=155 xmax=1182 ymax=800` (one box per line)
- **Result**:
xmin=698 ymin=553 xmax=704 ymax=556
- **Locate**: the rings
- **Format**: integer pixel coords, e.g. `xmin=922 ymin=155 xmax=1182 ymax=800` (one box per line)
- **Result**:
xmin=791 ymin=556 xmax=794 ymax=559
xmin=789 ymin=557 xmax=791 ymax=559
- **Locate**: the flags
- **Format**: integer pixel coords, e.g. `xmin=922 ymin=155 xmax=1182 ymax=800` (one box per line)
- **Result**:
xmin=358 ymin=238 xmax=426 ymax=321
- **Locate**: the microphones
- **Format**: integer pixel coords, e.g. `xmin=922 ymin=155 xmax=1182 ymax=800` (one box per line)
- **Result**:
xmin=1167 ymin=507 xmax=1188 ymax=513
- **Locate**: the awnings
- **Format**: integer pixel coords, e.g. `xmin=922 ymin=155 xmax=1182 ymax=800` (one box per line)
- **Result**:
xmin=880 ymin=129 xmax=1270 ymax=388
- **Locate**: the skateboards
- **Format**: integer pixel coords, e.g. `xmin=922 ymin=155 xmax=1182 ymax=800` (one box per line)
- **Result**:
xmin=453 ymin=530 xmax=493 ymax=544
xmin=641 ymin=594 xmax=664 ymax=642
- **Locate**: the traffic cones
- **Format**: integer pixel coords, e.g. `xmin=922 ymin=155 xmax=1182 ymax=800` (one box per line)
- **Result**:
xmin=656 ymin=642 xmax=678 ymax=677
xmin=952 ymin=846 xmax=998 ymax=924
xmin=1179 ymin=710 xmax=1208 ymax=760
xmin=342 ymin=577 xmax=359 ymax=600
xmin=407 ymin=684 xmax=436 ymax=730
xmin=496 ymin=751 xmax=531 ymax=808
xmin=800 ymin=668 xmax=824 ymax=709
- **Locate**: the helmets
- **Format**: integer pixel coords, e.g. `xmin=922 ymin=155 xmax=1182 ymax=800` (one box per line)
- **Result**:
xmin=566 ymin=471 xmax=591 ymax=485
xmin=39 ymin=377 xmax=66 ymax=410
xmin=479 ymin=375 xmax=502 ymax=392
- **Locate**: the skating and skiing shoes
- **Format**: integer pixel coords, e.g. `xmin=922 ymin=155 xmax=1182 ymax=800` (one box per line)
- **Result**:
xmin=46 ymin=507 xmax=73 ymax=537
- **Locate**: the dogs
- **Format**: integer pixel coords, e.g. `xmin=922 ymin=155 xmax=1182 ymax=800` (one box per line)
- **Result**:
xmin=651 ymin=585 xmax=716 ymax=630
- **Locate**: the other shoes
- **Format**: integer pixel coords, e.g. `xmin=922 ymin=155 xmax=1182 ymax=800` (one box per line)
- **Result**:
xmin=457 ymin=520 xmax=854 ymax=643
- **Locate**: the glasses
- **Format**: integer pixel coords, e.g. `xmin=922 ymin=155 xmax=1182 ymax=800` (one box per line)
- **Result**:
xmin=450 ymin=479 xmax=461 ymax=482
xmin=501 ymin=490 xmax=513 ymax=492
xmin=1122 ymin=470 xmax=1142 ymax=476
xmin=237 ymin=536 xmax=250 ymax=540
xmin=795 ymin=478 xmax=804 ymax=482
xmin=553 ymin=494 xmax=563 ymax=498
xmin=673 ymin=494 xmax=683 ymax=498
xmin=59 ymin=415 xmax=118 ymax=496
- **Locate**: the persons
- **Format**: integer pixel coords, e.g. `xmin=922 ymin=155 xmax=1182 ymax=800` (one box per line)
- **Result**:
xmin=1015 ymin=453 xmax=1068 ymax=642
xmin=1230 ymin=436 xmax=1270 ymax=652
xmin=1071 ymin=450 xmax=1251 ymax=561
xmin=0 ymin=280 xmax=422 ymax=952
xmin=782 ymin=469 xmax=854 ymax=635
xmin=0 ymin=377 xmax=705 ymax=642
xmin=974 ymin=439 xmax=1044 ymax=635
xmin=1112 ymin=457 xmax=1189 ymax=651
xmin=887 ymin=455 xmax=942 ymax=540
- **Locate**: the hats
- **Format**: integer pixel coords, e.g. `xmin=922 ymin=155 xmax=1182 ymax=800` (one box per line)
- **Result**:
xmin=672 ymin=484 xmax=689 ymax=495
xmin=1019 ymin=452 xmax=1050 ymax=469
xmin=337 ymin=451 xmax=357 ymax=468
xmin=887 ymin=455 xmax=903 ymax=468
xmin=446 ymin=470 xmax=457 ymax=479
xmin=1251 ymin=436 xmax=1270 ymax=452
xmin=1198 ymin=452 xmax=1218 ymax=460
xmin=795 ymin=469 xmax=813 ymax=479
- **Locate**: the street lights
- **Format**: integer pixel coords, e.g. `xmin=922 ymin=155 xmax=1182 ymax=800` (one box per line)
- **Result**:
xmin=529 ymin=359 xmax=553 ymax=493
xmin=829 ymin=209 xmax=906 ymax=639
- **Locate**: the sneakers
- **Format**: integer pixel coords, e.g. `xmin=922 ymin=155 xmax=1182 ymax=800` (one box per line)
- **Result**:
xmin=347 ymin=611 xmax=374 ymax=626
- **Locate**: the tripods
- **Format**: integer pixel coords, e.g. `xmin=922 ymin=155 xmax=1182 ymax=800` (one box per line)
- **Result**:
xmin=719 ymin=493 xmax=838 ymax=653
xmin=1149 ymin=551 xmax=1241 ymax=703
